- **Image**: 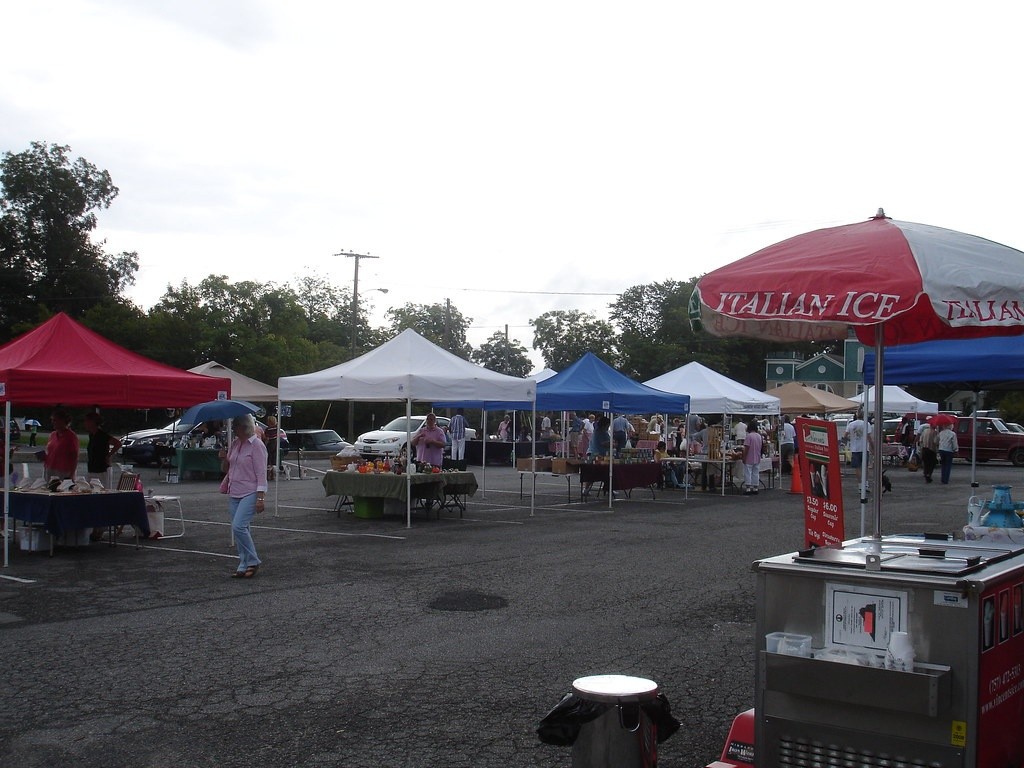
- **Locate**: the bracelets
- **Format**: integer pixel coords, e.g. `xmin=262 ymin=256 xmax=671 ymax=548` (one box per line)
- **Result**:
xmin=257 ymin=498 xmax=265 ymax=501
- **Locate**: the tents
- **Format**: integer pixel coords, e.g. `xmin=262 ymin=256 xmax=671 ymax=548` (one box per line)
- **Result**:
xmin=185 ymin=359 xmax=303 ymax=479
xmin=860 ymin=334 xmax=1024 ymax=541
xmin=846 ymin=383 xmax=938 ymax=435
xmin=276 ymin=327 xmax=537 ymax=526
xmin=641 ymin=360 xmax=783 ymax=496
xmin=759 ymin=379 xmax=860 ymax=491
xmin=525 ymin=367 xmax=558 ymax=386
xmin=0 ymin=312 xmax=235 ymax=566
xmin=428 ymin=400 xmax=570 ymax=470
xmin=481 ymin=350 xmax=691 ymax=507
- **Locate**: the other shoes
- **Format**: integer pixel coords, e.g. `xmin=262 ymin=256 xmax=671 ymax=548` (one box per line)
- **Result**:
xmin=856 ymin=489 xmax=861 ymax=494
xmin=940 ymin=479 xmax=949 ymax=484
xmin=743 ymin=491 xmax=752 ymax=495
xmin=31 ymin=446 xmax=36 ymax=448
xmin=925 ymin=475 xmax=933 ymax=483
xmin=27 ymin=444 xmax=30 ymax=447
xmin=612 ymin=491 xmax=619 ymax=496
xmin=865 ymin=485 xmax=871 ymax=493
xmin=581 ymin=488 xmax=591 ymax=495
xmin=752 ymin=490 xmax=758 ymax=494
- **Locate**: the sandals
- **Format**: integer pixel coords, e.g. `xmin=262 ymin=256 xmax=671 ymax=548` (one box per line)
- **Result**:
xmin=231 ymin=571 xmax=244 ymax=578
xmin=244 ymin=566 xmax=258 ymax=578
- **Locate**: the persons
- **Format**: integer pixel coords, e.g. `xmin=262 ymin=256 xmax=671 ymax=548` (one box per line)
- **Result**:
xmin=0 ymin=430 xmax=17 ymax=538
xmin=842 ymin=409 xmax=875 ymax=490
xmin=81 ymin=412 xmax=122 ymax=541
xmin=896 ymin=417 xmax=910 ymax=447
xmin=219 ymin=415 xmax=269 ymax=578
xmin=36 ymin=408 xmax=80 ymax=488
xmin=411 ymin=409 xmax=796 ymax=494
xmin=27 ymin=425 xmax=37 ymax=448
xmin=197 ymin=418 xmax=223 ymax=446
xmin=914 ymin=415 xmax=939 ymax=481
xmin=933 ymin=423 xmax=959 ymax=484
xmin=263 ymin=415 xmax=291 ymax=469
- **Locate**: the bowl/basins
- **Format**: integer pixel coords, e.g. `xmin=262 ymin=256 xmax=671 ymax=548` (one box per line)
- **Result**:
xmin=348 ymin=464 xmax=356 ymax=472
xmin=368 ymin=462 xmax=373 ymax=471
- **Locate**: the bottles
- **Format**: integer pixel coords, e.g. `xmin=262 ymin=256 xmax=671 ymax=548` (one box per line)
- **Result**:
xmin=166 ymin=472 xmax=178 ymax=484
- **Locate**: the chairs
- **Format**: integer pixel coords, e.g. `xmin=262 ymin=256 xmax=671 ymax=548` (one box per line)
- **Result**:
xmin=114 ymin=473 xmax=143 ymax=538
xmin=440 ymin=458 xmax=468 ymax=512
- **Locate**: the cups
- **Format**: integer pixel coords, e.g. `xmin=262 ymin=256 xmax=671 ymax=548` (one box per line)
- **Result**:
xmin=358 ymin=466 xmax=367 ymax=473
xmin=406 ymin=464 xmax=415 ymax=473
xmin=887 ymin=630 xmax=913 ymax=672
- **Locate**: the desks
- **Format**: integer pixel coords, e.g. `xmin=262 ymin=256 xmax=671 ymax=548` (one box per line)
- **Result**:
xmin=518 ymin=471 xmax=579 ymax=503
xmin=882 ymin=446 xmax=906 ymax=466
xmin=549 ymin=440 xmax=571 ymax=458
xmin=171 ymin=447 xmax=285 ymax=483
xmin=659 ymin=456 xmax=775 ymax=498
xmin=323 ymin=468 xmax=480 ymax=519
xmin=465 ymin=440 xmax=551 ymax=467
xmin=1 ymin=488 xmax=146 ymax=556
xmin=580 ymin=463 xmax=661 ymax=504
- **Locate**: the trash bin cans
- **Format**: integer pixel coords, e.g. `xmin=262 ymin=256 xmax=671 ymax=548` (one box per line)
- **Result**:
xmin=571 ymin=674 xmax=659 ymax=768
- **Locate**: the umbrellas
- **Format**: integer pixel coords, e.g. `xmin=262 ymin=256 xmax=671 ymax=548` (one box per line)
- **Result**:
xmin=178 ymin=398 xmax=262 ymax=450
xmin=927 ymin=413 xmax=959 ymax=429
xmin=23 ymin=419 xmax=42 ymax=427
xmin=688 ymin=209 xmax=1024 ymax=537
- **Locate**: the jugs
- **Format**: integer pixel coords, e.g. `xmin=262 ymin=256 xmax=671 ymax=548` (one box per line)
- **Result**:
xmin=967 ymin=497 xmax=987 ymax=526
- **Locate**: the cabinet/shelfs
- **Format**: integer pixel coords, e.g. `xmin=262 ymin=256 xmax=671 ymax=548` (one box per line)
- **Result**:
xmin=144 ymin=494 xmax=186 ymax=540
xmin=636 ymin=440 xmax=675 ymax=456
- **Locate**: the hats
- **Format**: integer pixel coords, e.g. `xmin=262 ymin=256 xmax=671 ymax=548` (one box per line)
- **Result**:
xmin=51 ymin=405 xmax=70 ymax=423
xmin=504 ymin=415 xmax=510 ymax=420
xmin=943 ymin=424 xmax=953 ymax=430
xmin=658 ymin=415 xmax=664 ymax=422
xmin=673 ymin=418 xmax=681 ymax=424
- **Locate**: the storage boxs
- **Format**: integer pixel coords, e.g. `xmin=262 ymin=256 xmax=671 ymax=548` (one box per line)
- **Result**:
xmin=516 ymin=411 xmax=792 ymax=473
xmin=384 ymin=493 xmax=417 ymax=515
xmin=353 ymin=496 xmax=383 ymax=516
xmin=166 ymin=473 xmax=179 ymax=482
xmin=18 ymin=524 xmax=91 ymax=554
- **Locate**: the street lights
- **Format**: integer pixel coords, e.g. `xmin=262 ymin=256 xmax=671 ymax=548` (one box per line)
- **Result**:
xmin=351 ymin=288 xmax=389 ymax=359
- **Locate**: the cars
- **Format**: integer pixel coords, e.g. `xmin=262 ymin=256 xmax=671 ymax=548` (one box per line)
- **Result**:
xmin=936 ymin=417 xmax=1024 ymax=468
xmin=791 ymin=410 xmax=1024 ymax=463
xmin=353 ymin=415 xmax=478 ymax=464
xmin=115 ymin=419 xmax=290 ymax=468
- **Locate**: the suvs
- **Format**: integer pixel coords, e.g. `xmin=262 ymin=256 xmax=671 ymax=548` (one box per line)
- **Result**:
xmin=284 ymin=429 xmax=353 ymax=451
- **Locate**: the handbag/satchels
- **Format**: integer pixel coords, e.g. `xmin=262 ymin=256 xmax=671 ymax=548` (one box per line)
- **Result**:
xmin=219 ymin=474 xmax=230 ymax=493
